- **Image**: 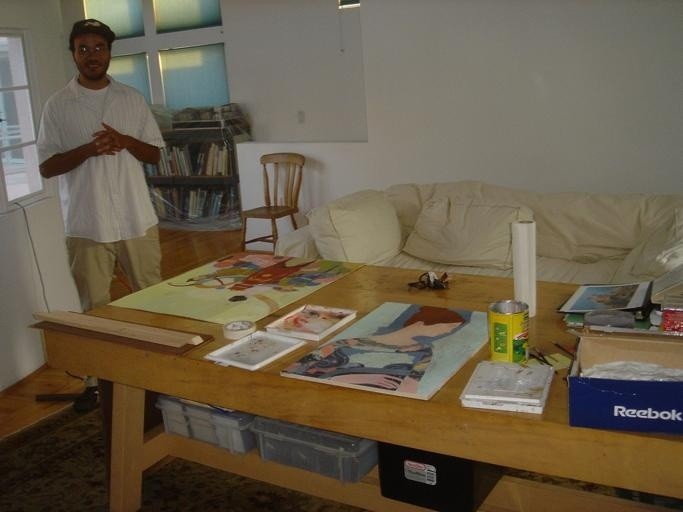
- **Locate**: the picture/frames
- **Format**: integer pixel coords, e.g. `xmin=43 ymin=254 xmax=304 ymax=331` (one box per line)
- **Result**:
xmin=202 ymin=330 xmax=306 ymax=370
xmin=264 ymin=303 xmax=356 ymax=342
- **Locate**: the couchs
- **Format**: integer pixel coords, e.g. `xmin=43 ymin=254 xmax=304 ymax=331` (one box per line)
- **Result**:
xmin=275 ymin=177 xmax=682 ymax=299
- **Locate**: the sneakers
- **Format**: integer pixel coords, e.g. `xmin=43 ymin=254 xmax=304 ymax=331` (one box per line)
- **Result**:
xmin=73 ymin=386 xmax=102 ymax=412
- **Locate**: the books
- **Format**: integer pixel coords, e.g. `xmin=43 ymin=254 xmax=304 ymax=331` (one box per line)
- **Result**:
xmin=650 ymin=263 xmax=683 ymax=336
xmin=143 ymin=139 xmax=236 ymax=218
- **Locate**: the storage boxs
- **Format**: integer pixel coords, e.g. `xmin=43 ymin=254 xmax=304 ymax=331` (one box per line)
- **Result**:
xmin=556 ymin=335 xmax=682 ymax=438
xmin=251 ymin=421 xmax=374 ymax=482
xmin=373 ymin=439 xmax=501 ymax=511
xmin=152 ymin=396 xmax=253 ymax=451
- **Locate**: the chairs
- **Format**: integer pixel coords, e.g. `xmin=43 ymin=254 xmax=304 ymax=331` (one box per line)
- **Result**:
xmin=237 ymin=151 xmax=305 ymax=254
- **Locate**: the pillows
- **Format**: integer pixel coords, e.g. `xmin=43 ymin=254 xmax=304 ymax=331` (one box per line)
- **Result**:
xmin=305 ymin=187 xmax=407 ymax=268
xmin=400 ymin=194 xmax=534 ymax=271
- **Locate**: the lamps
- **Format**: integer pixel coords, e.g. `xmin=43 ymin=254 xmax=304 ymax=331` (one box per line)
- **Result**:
xmin=335 ymin=0 xmax=359 ymax=10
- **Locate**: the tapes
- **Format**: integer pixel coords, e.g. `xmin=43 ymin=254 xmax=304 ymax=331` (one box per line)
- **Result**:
xmin=222 ymin=320 xmax=257 ymax=340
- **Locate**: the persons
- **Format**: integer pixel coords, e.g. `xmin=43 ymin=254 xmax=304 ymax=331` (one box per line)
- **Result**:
xmin=282 ymin=306 xmax=466 ymax=393
xmin=36 ymin=18 xmax=166 ymax=414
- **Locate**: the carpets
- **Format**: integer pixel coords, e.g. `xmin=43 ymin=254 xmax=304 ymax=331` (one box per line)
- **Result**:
xmin=0 ymin=395 xmax=368 ymax=510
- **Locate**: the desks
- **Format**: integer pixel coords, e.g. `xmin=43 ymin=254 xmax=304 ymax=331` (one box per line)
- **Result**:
xmin=35 ymin=252 xmax=683 ymax=512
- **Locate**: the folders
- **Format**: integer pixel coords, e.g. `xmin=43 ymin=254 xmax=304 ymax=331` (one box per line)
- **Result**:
xmin=556 ymin=281 xmax=654 ymax=320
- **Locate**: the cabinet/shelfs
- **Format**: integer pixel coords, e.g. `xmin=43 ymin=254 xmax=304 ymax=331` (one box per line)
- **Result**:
xmin=142 ymin=102 xmax=251 ymax=232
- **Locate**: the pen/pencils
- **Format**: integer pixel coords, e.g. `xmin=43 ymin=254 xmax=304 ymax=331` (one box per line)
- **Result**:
xmin=552 ymin=341 xmax=575 ymax=358
xmin=533 ymin=344 xmax=558 ymax=375
xmin=529 ymin=352 xmax=553 ymax=366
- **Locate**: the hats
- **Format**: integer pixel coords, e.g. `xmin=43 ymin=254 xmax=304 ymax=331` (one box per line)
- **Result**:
xmin=69 ymin=18 xmax=116 ymax=42
